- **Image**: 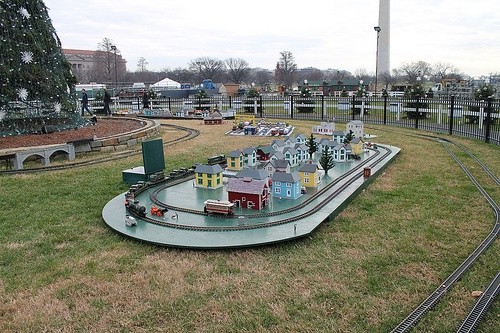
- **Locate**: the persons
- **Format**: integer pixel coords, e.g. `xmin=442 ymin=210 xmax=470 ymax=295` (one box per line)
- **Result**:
xmin=103 ymin=89 xmax=114 ymax=117
xmin=81 ymin=88 xmax=95 ymax=118
xmin=141 ymin=90 xmax=150 ymax=109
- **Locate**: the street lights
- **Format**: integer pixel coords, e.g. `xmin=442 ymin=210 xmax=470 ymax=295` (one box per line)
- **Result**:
xmin=111 ymin=46 xmax=118 ymax=97
xmin=373 ymin=27 xmax=381 ymax=106
xmin=196 ymin=61 xmax=204 ymax=90
